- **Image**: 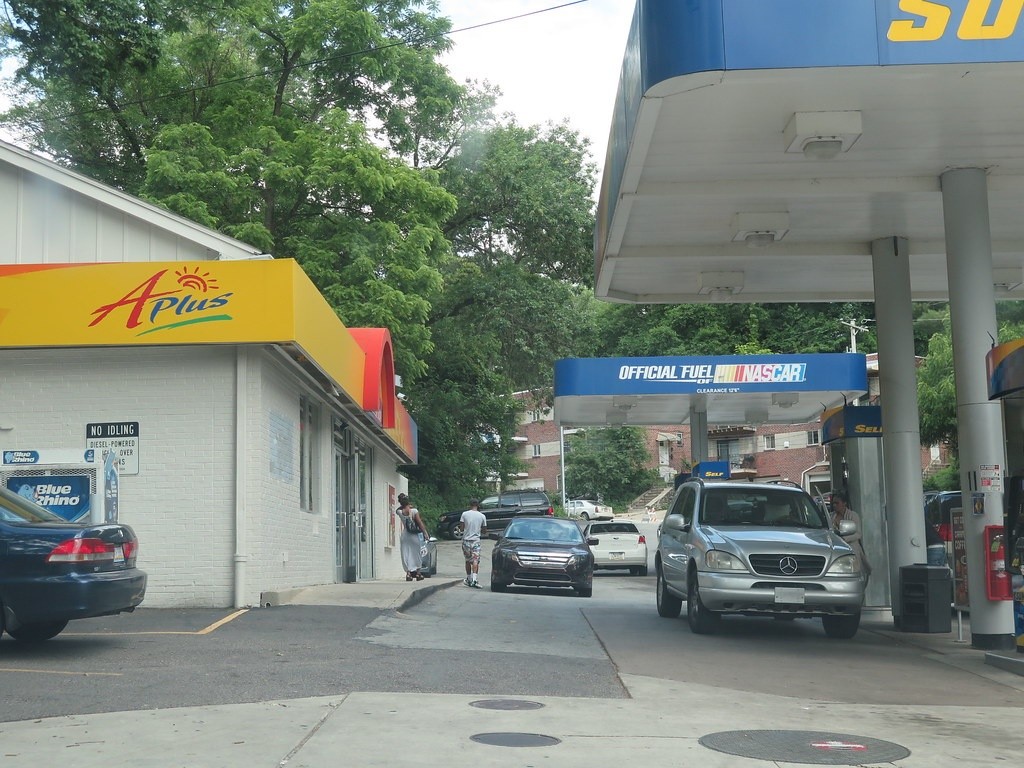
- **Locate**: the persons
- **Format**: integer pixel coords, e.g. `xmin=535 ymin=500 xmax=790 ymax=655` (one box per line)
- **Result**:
xmin=924 ymin=517 xmax=947 ymax=566
xmin=829 ymin=492 xmax=871 ymax=573
xmin=974 ymin=501 xmax=983 ymax=514
xmin=396 ymin=493 xmax=429 ymax=581
xmin=460 ymin=498 xmax=487 ymax=589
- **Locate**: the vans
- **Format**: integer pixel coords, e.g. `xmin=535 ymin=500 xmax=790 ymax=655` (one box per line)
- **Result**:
xmin=924 ymin=490 xmax=962 ymax=576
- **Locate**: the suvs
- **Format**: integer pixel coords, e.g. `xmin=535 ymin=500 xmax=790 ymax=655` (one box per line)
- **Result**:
xmin=436 ymin=489 xmax=554 ymax=540
xmin=654 ymin=477 xmax=866 ymax=639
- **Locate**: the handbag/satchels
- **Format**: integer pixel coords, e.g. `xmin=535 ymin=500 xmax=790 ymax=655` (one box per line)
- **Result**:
xmin=406 ymin=509 xmax=422 ymax=533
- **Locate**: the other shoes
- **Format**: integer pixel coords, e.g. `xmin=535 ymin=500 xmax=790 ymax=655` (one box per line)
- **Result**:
xmin=463 ymin=579 xmax=473 ymax=587
xmin=471 ymin=580 xmax=482 ymax=588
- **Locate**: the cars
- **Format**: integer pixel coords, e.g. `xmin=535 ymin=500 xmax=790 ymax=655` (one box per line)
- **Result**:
xmin=0 ymin=484 xmax=149 ymax=643
xmin=565 ymin=500 xmax=613 ymax=521
xmin=489 ymin=515 xmax=599 ymax=598
xmin=728 ymin=500 xmax=754 ymax=513
xmin=583 ymin=521 xmax=647 ymax=576
xmin=418 ymin=532 xmax=439 ymax=578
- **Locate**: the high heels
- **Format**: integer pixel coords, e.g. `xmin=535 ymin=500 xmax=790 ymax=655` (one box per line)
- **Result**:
xmin=406 ymin=574 xmax=413 ymax=581
xmin=416 ymin=573 xmax=424 ymax=581
